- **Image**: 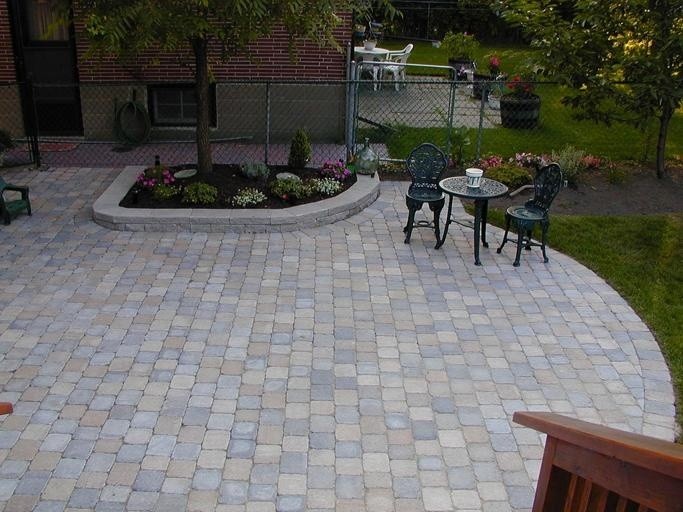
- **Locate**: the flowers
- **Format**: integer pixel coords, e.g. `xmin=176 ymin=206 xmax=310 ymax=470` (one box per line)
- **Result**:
xmin=136 ymin=169 xmax=176 ymax=190
xmin=507 ymin=76 xmax=532 ymax=98
xmin=321 ymin=159 xmax=350 ymax=182
xmin=488 ymin=55 xmax=500 ymax=76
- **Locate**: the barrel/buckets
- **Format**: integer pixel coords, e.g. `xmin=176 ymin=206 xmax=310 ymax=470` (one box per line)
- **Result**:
xmin=466 ymin=168 xmax=483 ymax=187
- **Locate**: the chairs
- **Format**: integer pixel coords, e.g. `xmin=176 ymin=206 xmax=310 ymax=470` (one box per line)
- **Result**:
xmin=354 ymin=44 xmax=413 ymax=92
xmin=0 ymin=178 xmax=32 ymax=225
xmin=496 ymin=161 xmax=564 ymax=266
xmin=403 ymin=142 xmax=450 ymax=246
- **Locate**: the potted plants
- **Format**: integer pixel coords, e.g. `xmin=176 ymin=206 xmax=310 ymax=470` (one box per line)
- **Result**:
xmin=361 ymin=31 xmax=377 ymax=51
xmin=441 ymin=34 xmax=479 ymax=82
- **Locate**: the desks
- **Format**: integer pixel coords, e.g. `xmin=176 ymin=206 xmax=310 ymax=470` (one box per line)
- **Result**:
xmin=434 ymin=176 xmax=509 ymax=266
